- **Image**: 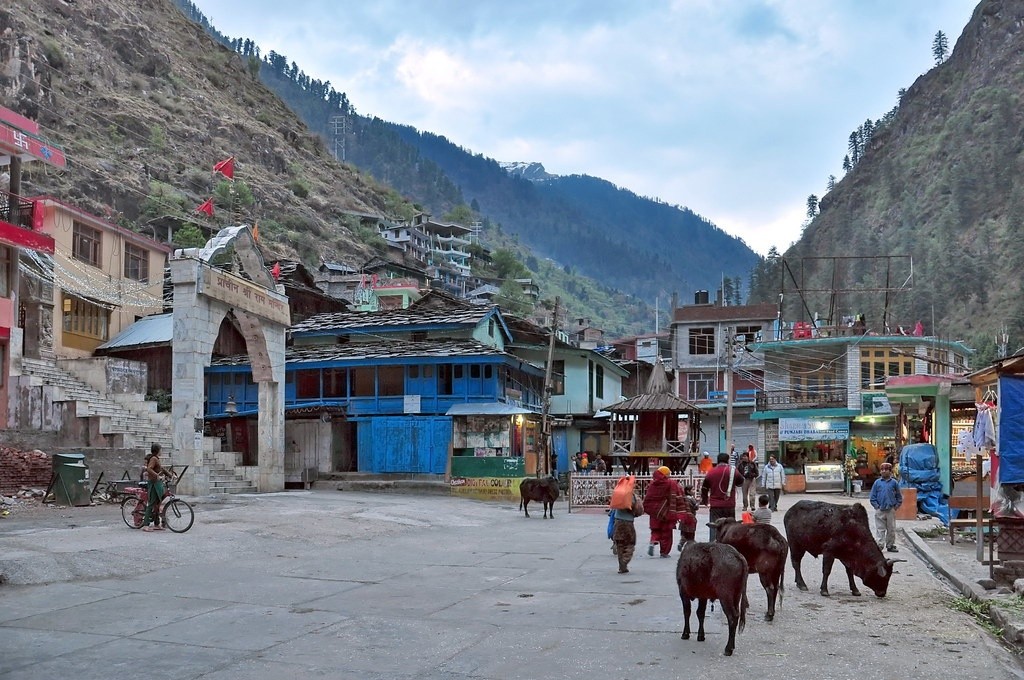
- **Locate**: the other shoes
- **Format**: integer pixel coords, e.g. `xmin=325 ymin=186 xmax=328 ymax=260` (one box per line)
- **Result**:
xmin=152 ymin=526 xmax=164 ymax=530
xmin=742 ymin=507 xmax=747 ymax=511
xmin=751 ymin=506 xmax=755 ymax=511
xmin=677 ymin=544 xmax=682 ymax=552
xmin=647 ymin=545 xmax=654 ymax=556
xmin=887 ymin=547 xmax=899 ymax=552
xmin=661 ymin=553 xmax=671 ymax=558
xmin=142 ymin=526 xmax=153 ymax=532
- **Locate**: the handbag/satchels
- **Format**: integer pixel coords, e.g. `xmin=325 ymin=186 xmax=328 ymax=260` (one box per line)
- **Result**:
xmin=610 ymin=476 xmax=635 ymax=510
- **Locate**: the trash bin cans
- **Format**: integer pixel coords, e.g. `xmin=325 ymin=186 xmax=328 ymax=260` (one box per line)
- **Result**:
xmin=52 ymin=454 xmax=91 ymax=506
xmin=895 ymin=488 xmax=917 ymax=520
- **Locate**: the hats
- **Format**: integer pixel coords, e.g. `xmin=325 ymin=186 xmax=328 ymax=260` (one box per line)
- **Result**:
xmin=703 ymin=452 xmax=709 ymax=455
xmin=658 ymin=466 xmax=670 ymax=476
xmin=880 ymin=463 xmax=893 ymax=470
xmin=582 ymin=453 xmax=587 ymax=458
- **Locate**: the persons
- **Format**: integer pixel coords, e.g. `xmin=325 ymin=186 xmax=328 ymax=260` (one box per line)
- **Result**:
xmin=699 ymin=451 xmax=712 ymax=474
xmin=751 ymin=494 xmax=771 ymax=525
xmin=701 ymin=453 xmax=745 ymax=542
xmin=730 ymin=444 xmax=739 ymax=467
xmin=788 ymin=447 xmax=900 ymax=479
xmin=870 ymin=462 xmax=902 ymax=553
xmin=613 ymin=477 xmax=644 ymax=573
xmin=739 ymin=453 xmax=759 ymax=512
xmin=762 ymin=454 xmax=785 ymax=512
xmin=677 ymin=485 xmax=698 ymax=552
xmin=134 ymin=443 xmax=177 ymax=531
xmin=550 ymin=452 xmax=613 ymax=481
xmin=747 ymin=444 xmax=757 ymax=462
xmin=644 ymin=466 xmax=684 ymax=559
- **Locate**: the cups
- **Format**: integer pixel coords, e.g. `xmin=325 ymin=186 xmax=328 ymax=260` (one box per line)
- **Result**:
xmin=968 ymin=512 xmax=972 ymax=520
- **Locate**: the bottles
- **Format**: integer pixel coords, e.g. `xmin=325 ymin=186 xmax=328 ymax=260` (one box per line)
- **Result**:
xmin=953 ymin=416 xmax=975 ymax=466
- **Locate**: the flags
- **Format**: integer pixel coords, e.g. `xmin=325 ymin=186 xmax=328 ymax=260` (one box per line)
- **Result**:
xmin=272 ymin=263 xmax=279 ymax=280
xmin=253 ymin=222 xmax=258 ymax=241
xmin=196 ymin=197 xmax=213 ymax=216
xmin=213 ymin=156 xmax=234 ymax=181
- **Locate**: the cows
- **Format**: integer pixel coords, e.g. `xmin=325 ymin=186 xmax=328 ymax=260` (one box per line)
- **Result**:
xmin=706 ymin=517 xmax=788 ymax=621
xmin=676 ymin=535 xmax=749 ymax=656
xmin=784 ymin=500 xmax=907 ymax=597
xmin=519 ymin=476 xmax=559 ymax=519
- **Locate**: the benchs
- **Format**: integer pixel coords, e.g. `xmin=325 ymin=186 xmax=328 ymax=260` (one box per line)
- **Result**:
xmin=735 ymin=388 xmax=761 ymax=401
xmin=707 ymin=390 xmax=729 ymax=403
xmin=948 ymin=495 xmax=992 ymax=545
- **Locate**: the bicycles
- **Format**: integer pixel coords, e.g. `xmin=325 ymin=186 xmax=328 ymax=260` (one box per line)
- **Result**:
xmin=119 ymin=471 xmax=194 ymax=533
